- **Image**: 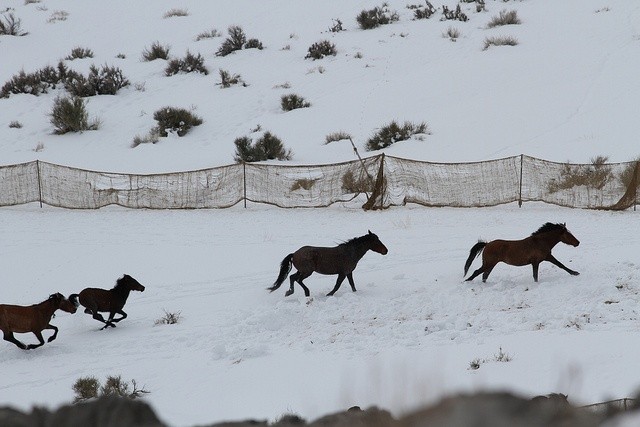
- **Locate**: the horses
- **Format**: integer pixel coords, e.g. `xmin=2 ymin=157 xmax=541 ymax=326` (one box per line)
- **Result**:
xmin=463 ymin=222 xmax=580 ymax=282
xmin=68 ymin=274 xmax=146 ymax=330
xmin=265 ymin=230 xmax=388 ymax=297
xmin=0 ymin=292 xmax=77 ymax=350
